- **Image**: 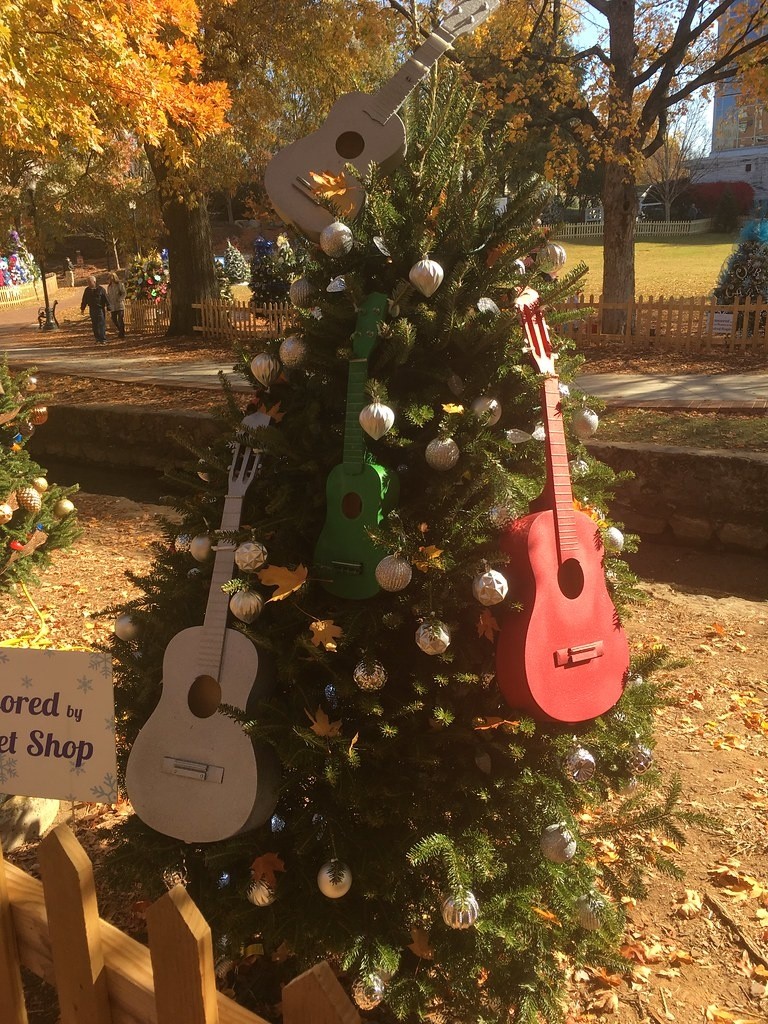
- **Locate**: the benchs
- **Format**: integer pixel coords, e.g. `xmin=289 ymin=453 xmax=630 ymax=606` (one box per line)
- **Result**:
xmin=37 ymin=299 xmax=60 ymax=329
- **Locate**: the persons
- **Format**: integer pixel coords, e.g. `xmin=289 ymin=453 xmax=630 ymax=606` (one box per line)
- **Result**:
xmin=107 ymin=273 xmax=127 ymax=338
xmin=689 ymin=203 xmax=703 ymax=220
xmin=80 ymin=276 xmax=111 ymax=345
xmin=525 ymin=227 xmax=557 ymax=282
xmin=63 ymin=256 xmax=74 ymax=287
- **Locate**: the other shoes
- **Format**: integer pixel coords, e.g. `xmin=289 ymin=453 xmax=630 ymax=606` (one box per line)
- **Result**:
xmin=96 ymin=339 xmax=107 ymax=344
xmin=118 ymin=333 xmax=124 ymax=338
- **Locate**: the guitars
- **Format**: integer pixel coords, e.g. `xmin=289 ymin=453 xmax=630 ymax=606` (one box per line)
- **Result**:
xmin=121 ymin=407 xmax=288 ymax=847
xmin=498 ymin=287 xmax=635 ymax=727
xmin=311 ymin=286 xmax=409 ymax=605
